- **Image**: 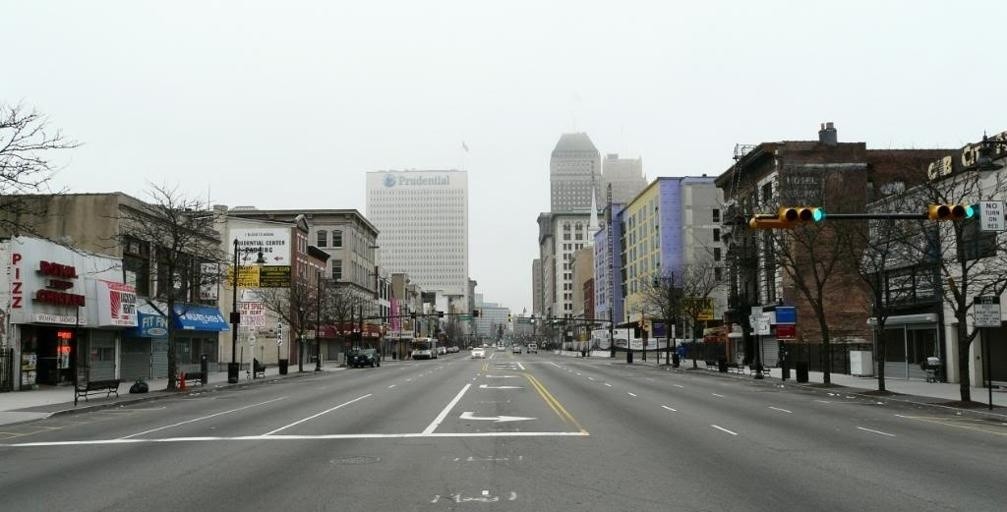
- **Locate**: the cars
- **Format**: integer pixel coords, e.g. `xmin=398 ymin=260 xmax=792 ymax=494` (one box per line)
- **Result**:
xmin=410 ymin=337 xmax=545 ymax=361
xmin=350 ymin=346 xmax=382 ymax=370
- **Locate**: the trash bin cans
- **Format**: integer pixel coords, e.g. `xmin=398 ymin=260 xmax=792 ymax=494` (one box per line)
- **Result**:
xmin=278 ymin=359 xmax=288 ymax=375
xmin=796 ymin=360 xmax=809 ymax=383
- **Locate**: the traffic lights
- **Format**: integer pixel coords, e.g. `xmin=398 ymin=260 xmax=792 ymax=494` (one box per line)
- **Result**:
xmin=927 ymin=201 xmax=981 ymax=224
xmin=777 ymin=204 xmax=827 ymax=230
xmin=506 ymin=312 xmax=513 ymax=323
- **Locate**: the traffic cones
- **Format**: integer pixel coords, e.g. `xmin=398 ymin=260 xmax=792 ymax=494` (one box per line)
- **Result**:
xmin=175 ymin=372 xmax=188 ymax=391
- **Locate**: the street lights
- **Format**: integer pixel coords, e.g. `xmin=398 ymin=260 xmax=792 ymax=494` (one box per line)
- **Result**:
xmin=226 ymin=238 xmax=267 ymax=385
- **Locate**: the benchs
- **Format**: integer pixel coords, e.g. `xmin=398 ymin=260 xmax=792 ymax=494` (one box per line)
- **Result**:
xmin=76 ymin=379 xmax=120 ymax=402
xmin=176 ymin=372 xmax=205 ymax=386
xmin=706 ymin=360 xmax=771 ymax=379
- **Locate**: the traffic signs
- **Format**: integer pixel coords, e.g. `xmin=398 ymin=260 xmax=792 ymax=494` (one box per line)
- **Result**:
xmin=980 ymin=201 xmax=1005 ymax=232
xmin=456 ymin=316 xmax=472 ymax=320
